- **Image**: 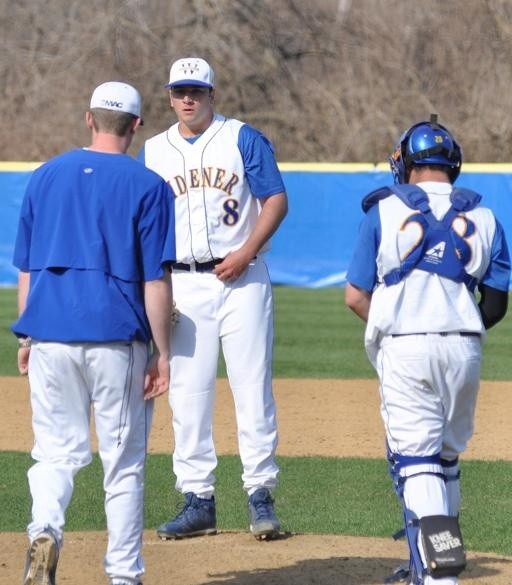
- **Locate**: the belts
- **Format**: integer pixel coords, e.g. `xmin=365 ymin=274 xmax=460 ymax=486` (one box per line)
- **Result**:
xmin=391 ymin=332 xmax=481 ymax=336
xmin=172 ymin=255 xmax=257 ymax=270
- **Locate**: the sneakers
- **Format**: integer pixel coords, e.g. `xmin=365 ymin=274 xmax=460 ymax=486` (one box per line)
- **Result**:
xmin=22 ymin=527 xmax=60 ymax=584
xmin=245 ymin=487 xmax=280 ymax=540
xmin=154 ymin=490 xmax=217 ymax=540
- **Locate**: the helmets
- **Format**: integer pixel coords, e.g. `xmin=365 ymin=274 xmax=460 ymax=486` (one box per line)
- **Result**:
xmin=391 ymin=120 xmax=462 ymax=186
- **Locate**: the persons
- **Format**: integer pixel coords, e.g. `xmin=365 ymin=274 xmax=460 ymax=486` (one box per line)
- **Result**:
xmin=135 ymin=57 xmax=288 ymax=540
xmin=11 ymin=81 xmax=177 ymax=585
xmin=344 ymin=121 xmax=511 ymax=585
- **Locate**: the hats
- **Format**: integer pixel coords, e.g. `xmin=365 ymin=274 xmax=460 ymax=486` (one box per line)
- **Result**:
xmin=86 ymin=79 xmax=148 ymax=126
xmin=162 ymin=57 xmax=217 ymax=96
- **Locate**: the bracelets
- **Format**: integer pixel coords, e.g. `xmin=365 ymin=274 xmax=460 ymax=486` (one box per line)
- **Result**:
xmin=18 ymin=341 xmax=31 ymax=347
